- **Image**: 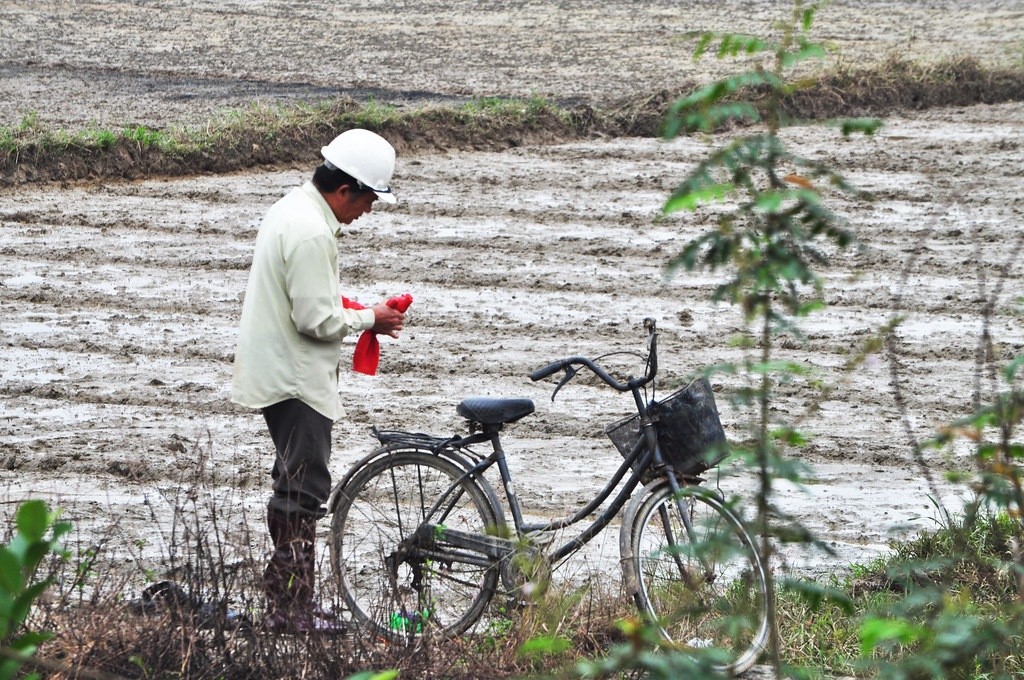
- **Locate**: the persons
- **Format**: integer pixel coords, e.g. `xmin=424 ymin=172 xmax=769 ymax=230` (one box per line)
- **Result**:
xmin=229 ymin=128 xmax=406 ymax=632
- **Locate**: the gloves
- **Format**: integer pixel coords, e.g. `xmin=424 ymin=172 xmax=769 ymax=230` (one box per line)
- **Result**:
xmin=350 ymin=298 xmax=400 ymax=376
xmin=342 ymin=294 xmax=413 ymax=313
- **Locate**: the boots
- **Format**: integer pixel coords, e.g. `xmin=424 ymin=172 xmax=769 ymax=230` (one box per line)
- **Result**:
xmin=272 ymin=516 xmax=347 ymax=632
xmin=258 ymin=515 xmax=337 ymax=618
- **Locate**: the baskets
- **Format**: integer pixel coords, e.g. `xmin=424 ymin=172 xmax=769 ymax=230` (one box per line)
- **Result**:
xmin=605 ymin=377 xmax=731 ymax=492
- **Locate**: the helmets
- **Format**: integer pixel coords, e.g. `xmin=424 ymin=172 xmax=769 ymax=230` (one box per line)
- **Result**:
xmin=320 ymin=128 xmax=397 ymax=204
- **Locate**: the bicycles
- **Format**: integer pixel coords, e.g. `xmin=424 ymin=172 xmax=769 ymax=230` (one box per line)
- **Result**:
xmin=327 ymin=314 xmax=774 ymax=679
